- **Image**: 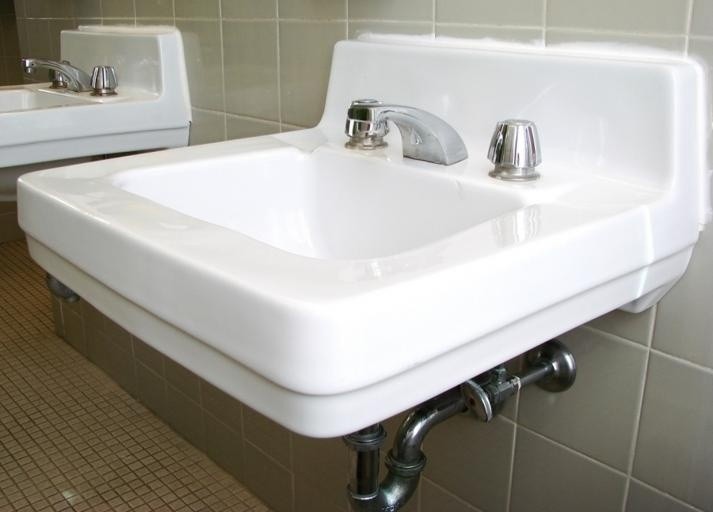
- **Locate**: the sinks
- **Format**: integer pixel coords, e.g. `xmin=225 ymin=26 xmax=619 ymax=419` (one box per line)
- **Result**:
xmin=19 ymin=127 xmax=667 ymax=441
xmin=0 ymin=82 xmax=161 ymax=169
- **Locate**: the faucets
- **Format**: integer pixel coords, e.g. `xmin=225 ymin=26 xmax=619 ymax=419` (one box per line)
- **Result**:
xmin=346 ymin=103 xmax=468 ymax=165
xmin=20 ymin=57 xmax=92 ymax=93
